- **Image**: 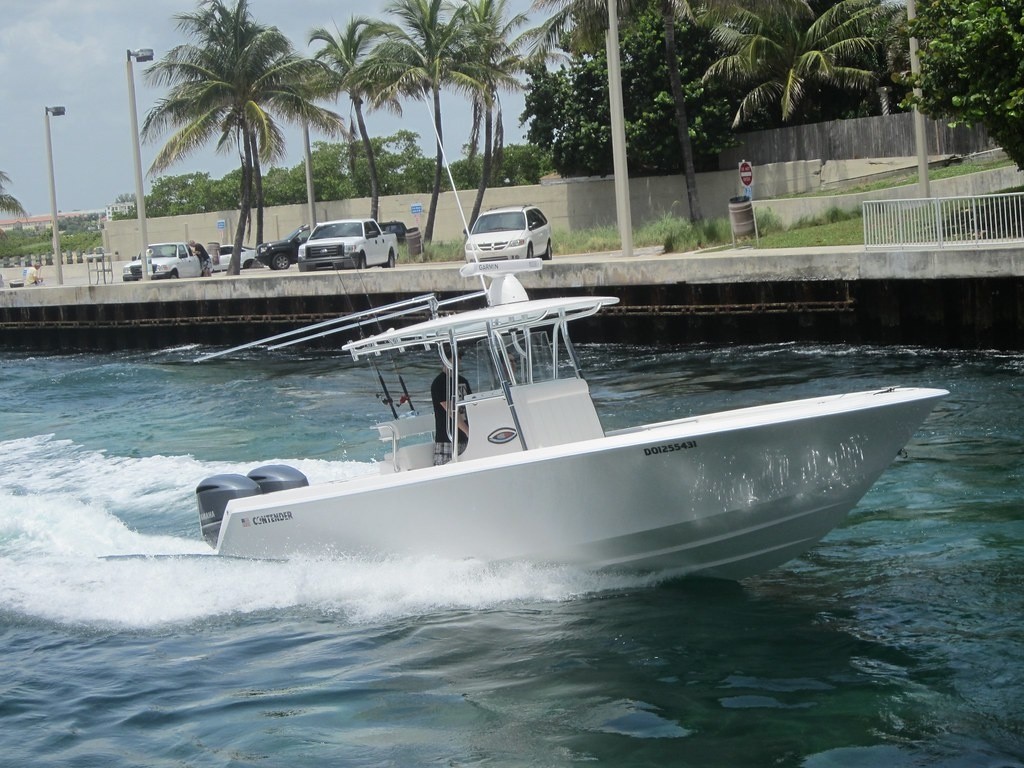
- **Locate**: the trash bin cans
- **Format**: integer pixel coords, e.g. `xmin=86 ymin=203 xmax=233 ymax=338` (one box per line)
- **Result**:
xmin=206 ymin=242 xmax=222 ymax=273
xmin=404 ymin=227 xmax=423 ymax=264
xmin=727 ymin=196 xmax=760 ymax=249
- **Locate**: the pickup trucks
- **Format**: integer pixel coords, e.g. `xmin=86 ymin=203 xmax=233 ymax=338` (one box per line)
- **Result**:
xmin=120 ymin=242 xmax=213 ymax=282
xmin=297 ymin=219 xmax=399 ymax=271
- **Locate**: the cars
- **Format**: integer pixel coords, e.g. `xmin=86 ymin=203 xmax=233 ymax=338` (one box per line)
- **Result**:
xmin=206 ymin=244 xmax=258 ymax=273
xmin=255 ymin=223 xmax=323 ymax=273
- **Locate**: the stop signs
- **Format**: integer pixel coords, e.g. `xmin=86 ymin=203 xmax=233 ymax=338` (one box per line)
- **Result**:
xmin=738 ymin=161 xmax=753 ymax=188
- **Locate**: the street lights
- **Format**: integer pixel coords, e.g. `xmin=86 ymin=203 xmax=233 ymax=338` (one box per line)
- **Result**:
xmin=45 ymin=105 xmax=66 ymax=286
xmin=126 ymin=48 xmax=155 ymax=282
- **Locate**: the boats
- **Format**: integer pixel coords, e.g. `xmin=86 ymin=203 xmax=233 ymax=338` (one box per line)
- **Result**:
xmin=96 ymin=83 xmax=947 ymax=598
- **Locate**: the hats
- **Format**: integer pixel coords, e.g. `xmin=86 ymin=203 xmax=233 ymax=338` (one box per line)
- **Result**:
xmin=444 ymin=349 xmax=464 ymax=359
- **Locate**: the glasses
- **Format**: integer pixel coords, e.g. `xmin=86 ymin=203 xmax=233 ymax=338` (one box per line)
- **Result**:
xmin=510 ymin=358 xmax=517 ymax=363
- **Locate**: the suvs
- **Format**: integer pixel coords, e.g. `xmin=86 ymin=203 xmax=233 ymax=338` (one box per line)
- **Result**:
xmin=464 ymin=204 xmax=553 ymax=262
xmin=376 ymin=220 xmax=407 ymax=250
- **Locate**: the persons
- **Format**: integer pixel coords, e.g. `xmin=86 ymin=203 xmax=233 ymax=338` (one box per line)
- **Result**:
xmin=26 ymin=263 xmax=47 ymax=287
xmin=429 ymin=350 xmax=472 ymax=466
xmin=188 ymin=240 xmax=213 ymax=277
xmin=492 ymin=351 xmax=521 ymax=438
